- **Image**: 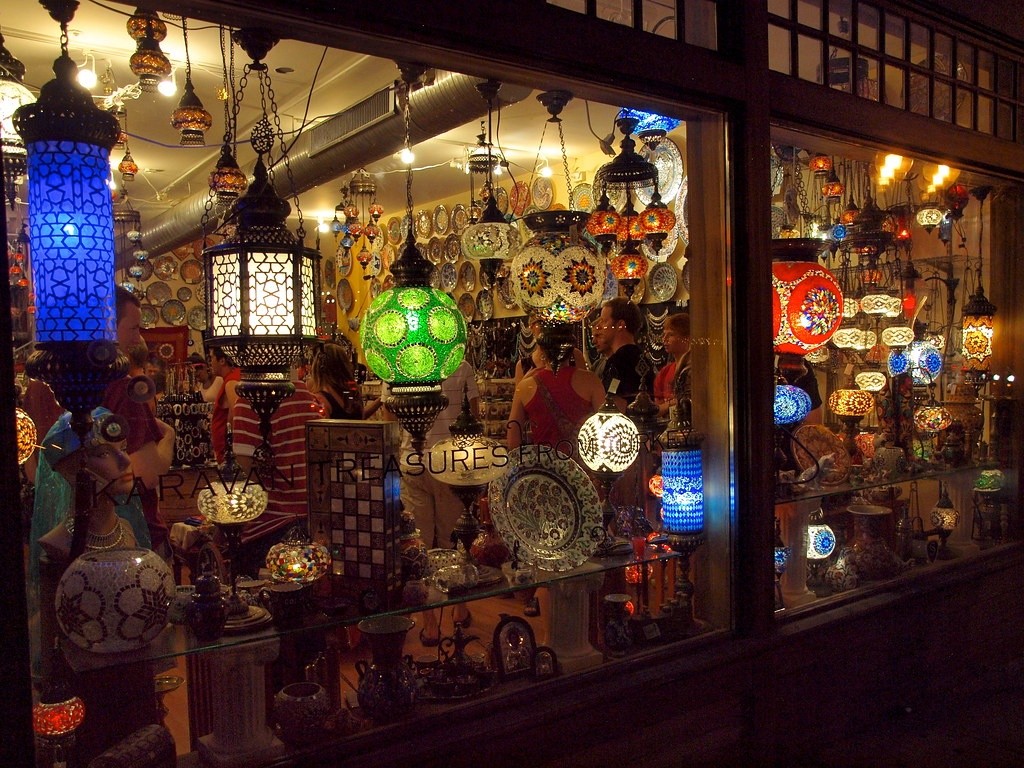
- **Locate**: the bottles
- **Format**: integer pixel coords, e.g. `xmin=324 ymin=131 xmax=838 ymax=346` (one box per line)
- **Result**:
xmin=604 ymin=593 xmax=633 ymax=655
xmin=354 ymin=614 xmax=416 ymax=726
xmin=845 ymin=504 xmax=904 ymax=585
xmin=190 ymin=563 xmax=226 ymax=642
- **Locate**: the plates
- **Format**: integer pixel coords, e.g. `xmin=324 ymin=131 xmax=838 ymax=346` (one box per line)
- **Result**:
xmin=324 ymin=135 xmax=688 ymax=324
xmin=125 ymin=236 xmax=217 ymax=331
xmin=791 ymin=423 xmax=851 ymax=487
xmin=487 ymin=444 xmax=604 ymax=573
xmin=224 ymin=605 xmax=273 ymax=635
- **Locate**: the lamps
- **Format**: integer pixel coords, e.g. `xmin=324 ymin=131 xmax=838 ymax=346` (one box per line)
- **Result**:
xmin=0 ymin=0 xmax=1024 ymax=768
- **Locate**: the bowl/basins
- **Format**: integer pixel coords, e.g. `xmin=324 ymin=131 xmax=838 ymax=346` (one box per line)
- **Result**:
xmin=55 ymin=547 xmax=178 ymax=654
xmin=272 ymin=681 xmax=329 ymax=749
xmin=872 ymin=446 xmax=906 ymax=481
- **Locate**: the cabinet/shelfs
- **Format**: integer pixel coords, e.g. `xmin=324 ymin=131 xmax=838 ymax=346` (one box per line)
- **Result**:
xmin=27 ymin=439 xmax=1024 ymax=768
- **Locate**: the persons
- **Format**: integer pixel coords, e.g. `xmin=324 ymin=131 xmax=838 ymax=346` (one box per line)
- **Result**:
xmin=23 ymin=288 xmax=693 ymax=531
xmin=36 ymin=407 xmax=136 ymax=559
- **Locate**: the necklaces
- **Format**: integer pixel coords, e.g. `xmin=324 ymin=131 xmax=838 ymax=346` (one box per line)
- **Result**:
xmin=66 ymin=514 xmax=128 ymax=553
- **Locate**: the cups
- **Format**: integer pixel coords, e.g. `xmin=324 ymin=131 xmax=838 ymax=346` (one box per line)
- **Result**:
xmin=259 ymin=582 xmax=305 ymax=631
xmin=236 ymin=578 xmax=267 ymax=596
